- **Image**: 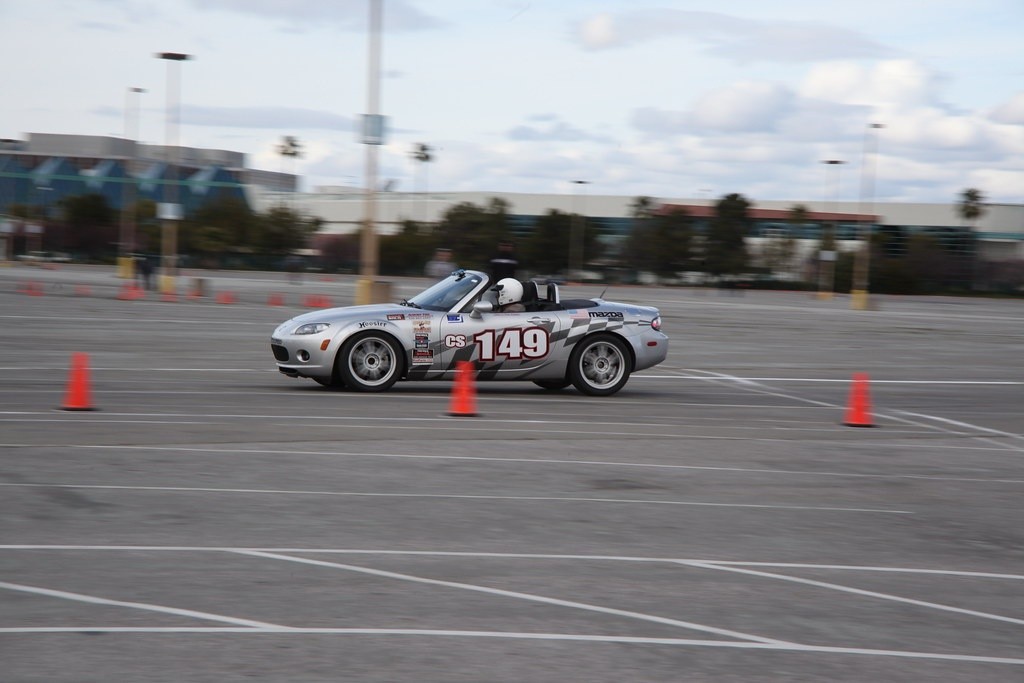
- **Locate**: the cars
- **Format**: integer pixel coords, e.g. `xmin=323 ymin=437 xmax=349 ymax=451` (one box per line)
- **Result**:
xmin=269 ymin=269 xmax=668 ymax=398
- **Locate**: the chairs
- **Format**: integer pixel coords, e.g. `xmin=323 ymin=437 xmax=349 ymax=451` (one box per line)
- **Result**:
xmin=521 ymin=280 xmax=563 ymax=311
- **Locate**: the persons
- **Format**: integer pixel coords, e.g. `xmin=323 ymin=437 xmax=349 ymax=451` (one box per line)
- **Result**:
xmin=491 ymin=277 xmax=526 ymax=312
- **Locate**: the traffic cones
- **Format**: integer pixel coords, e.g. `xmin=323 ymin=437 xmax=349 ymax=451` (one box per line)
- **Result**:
xmin=443 ymin=361 xmax=484 ymax=417
xmin=56 ymin=353 xmax=101 ymax=413
xmin=836 ymin=372 xmax=879 ymax=428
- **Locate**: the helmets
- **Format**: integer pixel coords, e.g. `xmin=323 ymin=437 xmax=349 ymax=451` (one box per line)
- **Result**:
xmin=491 ymin=278 xmax=523 ymax=306
